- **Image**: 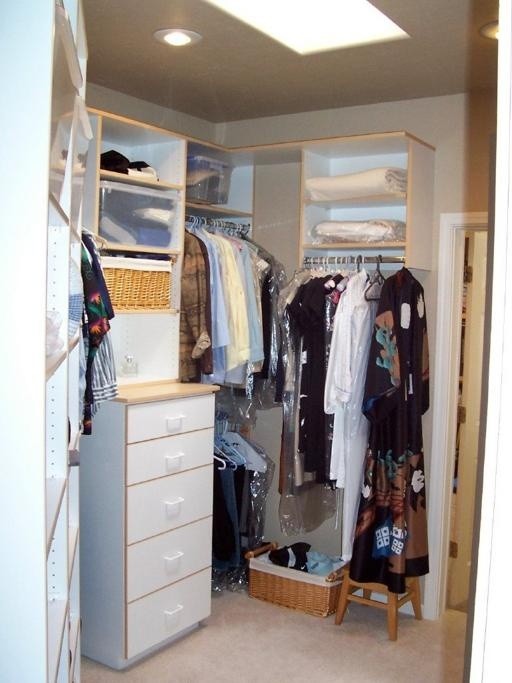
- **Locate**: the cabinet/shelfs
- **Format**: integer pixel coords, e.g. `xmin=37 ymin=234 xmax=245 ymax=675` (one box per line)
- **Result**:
xmin=63 ymin=105 xmax=435 ymax=384
xmin=76 ymin=379 xmax=222 ymax=671
xmin=2 ymin=0 xmax=83 ymax=680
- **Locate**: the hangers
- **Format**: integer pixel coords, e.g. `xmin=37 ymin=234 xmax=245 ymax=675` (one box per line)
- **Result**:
xmin=188 ymin=214 xmax=249 ymax=236
xmin=301 ymin=254 xmax=386 ymax=300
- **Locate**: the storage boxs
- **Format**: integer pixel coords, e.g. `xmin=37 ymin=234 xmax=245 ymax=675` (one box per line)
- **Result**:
xmin=187 ymin=154 xmax=234 ymax=203
xmin=99 ymin=179 xmax=182 ymax=246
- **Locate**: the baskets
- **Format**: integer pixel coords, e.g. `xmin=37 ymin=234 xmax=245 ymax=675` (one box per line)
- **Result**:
xmin=247 ymin=545 xmax=345 ymax=619
xmin=98 ymin=255 xmax=173 ymax=310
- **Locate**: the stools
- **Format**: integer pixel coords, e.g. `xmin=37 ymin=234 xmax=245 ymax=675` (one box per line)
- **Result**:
xmin=333 ymin=569 xmax=425 ymax=640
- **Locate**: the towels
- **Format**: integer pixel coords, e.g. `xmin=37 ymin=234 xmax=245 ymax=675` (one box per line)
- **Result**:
xmin=307 ymin=167 xmax=406 ymax=193
